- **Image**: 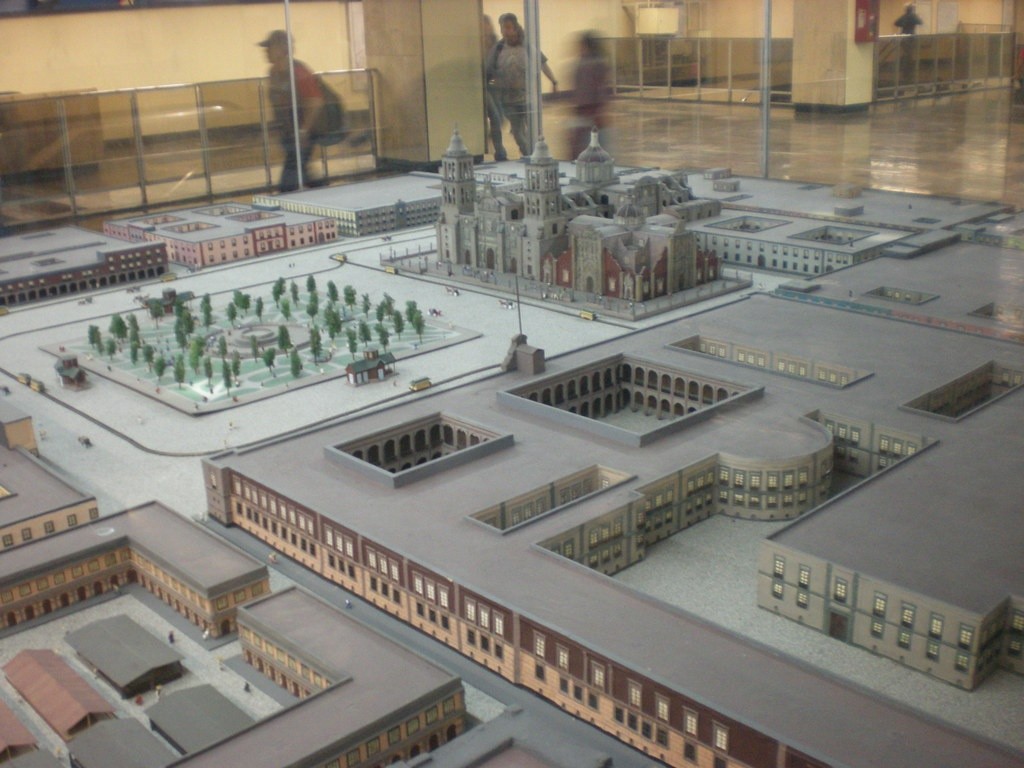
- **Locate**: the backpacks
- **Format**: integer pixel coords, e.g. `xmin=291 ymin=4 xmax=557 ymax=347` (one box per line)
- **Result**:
xmin=291 ymin=60 xmax=346 ymax=146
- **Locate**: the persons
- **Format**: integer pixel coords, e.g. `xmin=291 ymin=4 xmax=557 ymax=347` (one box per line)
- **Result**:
xmin=260 ymin=29 xmax=345 ymax=192
xmin=572 ymin=32 xmax=614 ymax=160
xmin=895 ymin=2 xmax=923 ymax=34
xmin=483 ymin=14 xmax=559 ymax=159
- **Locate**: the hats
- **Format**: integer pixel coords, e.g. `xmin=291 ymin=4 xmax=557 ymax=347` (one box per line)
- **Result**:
xmin=258 ymin=30 xmax=291 ymax=46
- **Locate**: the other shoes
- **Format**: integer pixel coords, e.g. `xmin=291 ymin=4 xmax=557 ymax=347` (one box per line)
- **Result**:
xmin=496 ymin=157 xmax=508 ymax=162
